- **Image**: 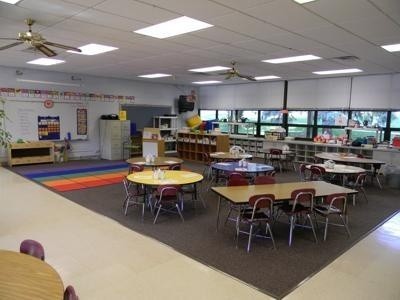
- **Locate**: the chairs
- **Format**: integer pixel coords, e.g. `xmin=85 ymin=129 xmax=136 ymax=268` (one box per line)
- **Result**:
xmin=122 ymin=137 xmax=388 ymax=255
xmin=64 ymin=285 xmax=78 ymax=300
xmin=19 ymin=238 xmax=44 ymax=263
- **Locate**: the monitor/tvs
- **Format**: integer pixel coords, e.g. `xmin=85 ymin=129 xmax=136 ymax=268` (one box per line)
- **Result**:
xmin=388 ymin=132 xmax=400 ymax=149
xmin=218 ymin=122 xmax=235 ymax=133
xmin=233 ymin=124 xmax=258 ymax=136
xmin=285 ymin=126 xmax=315 ymax=139
xmin=348 ymin=130 xmax=386 ymax=144
xmin=234 ymin=110 xmax=260 ymax=123
xmin=315 ymin=109 xmax=349 ymax=128
xmin=349 ymin=109 xmax=387 ymax=129
xmin=198 ymin=109 xmax=217 ymax=121
xmin=388 ymin=110 xmax=400 ymax=130
xmin=257 ymin=125 xmax=286 ymax=141
xmin=314 ymin=127 xmax=348 ymax=143
xmin=285 ymin=109 xmax=316 ymax=125
xmin=258 ymin=111 xmax=285 ymax=124
xmin=216 ymin=109 xmax=233 ymax=121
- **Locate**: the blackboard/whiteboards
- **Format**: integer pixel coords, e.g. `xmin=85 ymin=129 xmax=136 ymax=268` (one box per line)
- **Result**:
xmin=3 ymin=100 xmax=89 ymax=144
xmin=121 ymin=104 xmax=172 ymax=131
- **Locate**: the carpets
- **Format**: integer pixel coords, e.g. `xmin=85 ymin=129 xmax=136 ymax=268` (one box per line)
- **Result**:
xmin=1 ymin=157 xmax=400 ymax=300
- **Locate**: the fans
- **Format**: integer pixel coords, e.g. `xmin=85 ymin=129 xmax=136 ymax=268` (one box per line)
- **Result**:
xmin=0 ymin=18 xmax=82 ymax=58
xmin=212 ymin=60 xmax=257 ymax=83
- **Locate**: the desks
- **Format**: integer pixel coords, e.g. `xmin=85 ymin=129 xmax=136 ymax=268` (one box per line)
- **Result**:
xmin=1 ymin=250 xmax=65 ymax=300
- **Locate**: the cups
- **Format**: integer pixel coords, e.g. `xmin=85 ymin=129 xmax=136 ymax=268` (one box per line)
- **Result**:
xmin=232 ymin=149 xmax=239 ymax=155
xmin=145 ymin=153 xmax=156 ymax=162
xmin=239 ymin=158 xmax=247 ymax=167
xmin=324 ymin=159 xmax=335 ymax=169
xmin=153 ymin=169 xmax=165 ymax=180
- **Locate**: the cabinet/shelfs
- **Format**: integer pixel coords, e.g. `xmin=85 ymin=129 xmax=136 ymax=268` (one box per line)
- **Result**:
xmin=153 ymin=116 xmax=177 ymax=155
xmin=98 ymin=117 xmax=132 ymax=161
xmin=178 ymin=131 xmax=230 ymax=165
xmin=6 ymin=140 xmax=56 ymax=168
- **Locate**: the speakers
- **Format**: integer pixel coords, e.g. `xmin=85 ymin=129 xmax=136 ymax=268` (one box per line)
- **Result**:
xmin=177 ymin=94 xmax=195 ymax=114
